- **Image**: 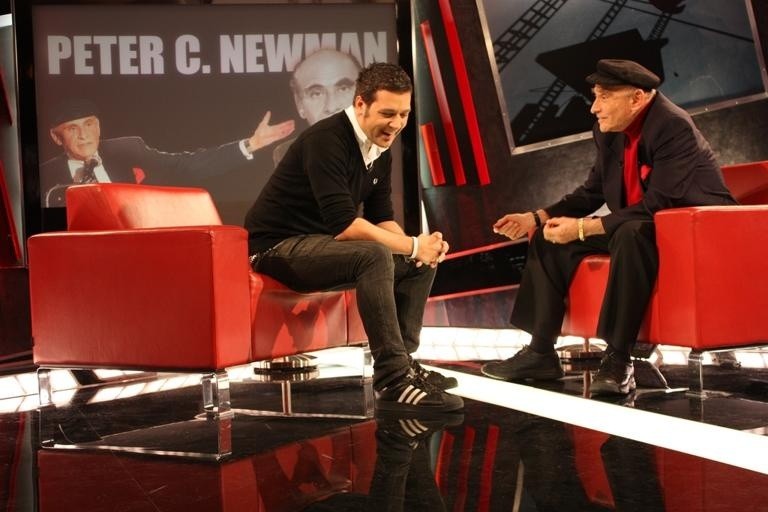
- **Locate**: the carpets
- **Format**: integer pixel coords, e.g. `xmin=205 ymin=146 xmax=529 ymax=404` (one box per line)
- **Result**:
xmin=81 ymin=158 xmax=99 ymax=184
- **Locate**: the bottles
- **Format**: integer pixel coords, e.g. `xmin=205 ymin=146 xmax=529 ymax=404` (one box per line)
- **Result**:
xmin=585 ymin=58 xmax=660 ymax=90
xmin=48 ymin=96 xmax=99 ymax=128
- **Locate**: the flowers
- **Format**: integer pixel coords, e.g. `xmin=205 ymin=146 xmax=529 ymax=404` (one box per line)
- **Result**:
xmin=81 ymin=155 xmax=103 ymax=183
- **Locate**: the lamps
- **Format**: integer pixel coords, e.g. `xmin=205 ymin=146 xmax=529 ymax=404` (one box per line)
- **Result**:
xmin=39 ymin=421 xmax=377 ymax=512
xmin=558 ymin=160 xmax=767 ymax=401
xmin=27 ymin=183 xmax=375 ymax=462
xmin=569 ymin=424 xmax=768 ymax=512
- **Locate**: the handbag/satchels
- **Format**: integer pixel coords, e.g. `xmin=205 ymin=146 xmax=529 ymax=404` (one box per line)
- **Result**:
xmin=578 ymin=218 xmax=587 ymax=242
xmin=532 ymin=211 xmax=542 ymax=231
xmin=405 ymin=236 xmax=418 ymax=264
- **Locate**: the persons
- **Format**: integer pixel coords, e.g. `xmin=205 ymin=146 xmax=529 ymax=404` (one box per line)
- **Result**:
xmin=497 ymin=400 xmax=667 ymax=512
xmin=242 ymin=61 xmax=466 ymax=413
xmin=481 ymin=60 xmax=738 ymax=397
xmin=368 ymin=412 xmax=471 ymax=511
xmin=271 ymin=45 xmax=366 ymax=169
xmin=38 ymin=99 xmax=295 ymax=209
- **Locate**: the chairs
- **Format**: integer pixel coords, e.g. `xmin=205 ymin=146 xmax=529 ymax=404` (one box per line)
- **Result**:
xmin=411 ymin=360 xmax=458 ymax=390
xmin=588 ymin=351 xmax=637 ymax=395
xmin=374 ymin=367 xmax=465 ymax=413
xmin=481 ymin=344 xmax=565 ymax=382
